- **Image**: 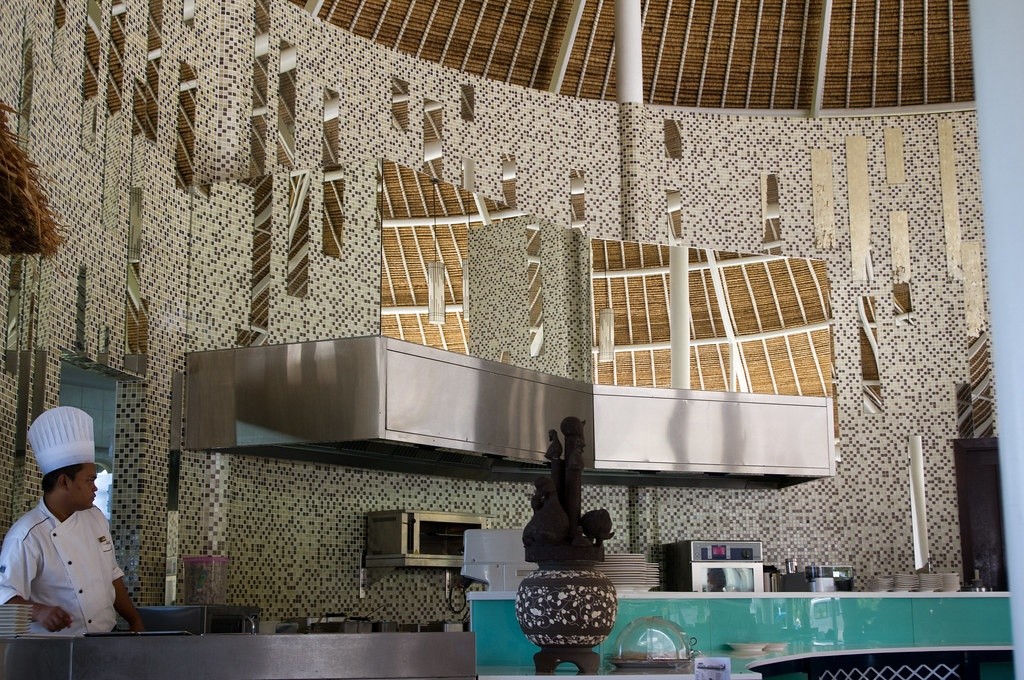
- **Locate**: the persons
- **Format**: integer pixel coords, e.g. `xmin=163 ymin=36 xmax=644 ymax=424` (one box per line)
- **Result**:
xmin=0 ymin=406 xmax=148 ymax=637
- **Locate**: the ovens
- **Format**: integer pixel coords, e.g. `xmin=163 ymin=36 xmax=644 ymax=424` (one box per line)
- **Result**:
xmin=663 ymin=536 xmax=764 ymax=593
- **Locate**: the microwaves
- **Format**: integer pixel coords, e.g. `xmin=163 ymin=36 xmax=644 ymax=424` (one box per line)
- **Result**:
xmin=137 ymin=602 xmax=260 ymax=636
xmin=365 ymin=509 xmax=494 ymax=572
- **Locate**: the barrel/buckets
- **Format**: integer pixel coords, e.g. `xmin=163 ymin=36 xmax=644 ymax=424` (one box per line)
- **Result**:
xmin=181 ymin=555 xmax=230 ymax=606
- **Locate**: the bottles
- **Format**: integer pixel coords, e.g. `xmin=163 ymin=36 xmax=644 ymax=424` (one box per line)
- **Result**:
xmin=786 ymin=557 xmax=798 ymax=574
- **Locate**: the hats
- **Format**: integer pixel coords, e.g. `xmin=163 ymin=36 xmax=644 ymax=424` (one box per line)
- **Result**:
xmin=27 ymin=406 xmax=95 ymax=476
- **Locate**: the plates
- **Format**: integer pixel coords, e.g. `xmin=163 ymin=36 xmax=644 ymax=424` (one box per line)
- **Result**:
xmin=727 ymin=643 xmax=768 ymax=651
xmin=762 ymin=643 xmax=791 ymax=651
xmin=596 ymin=553 xmax=660 ymax=593
xmin=870 ymin=572 xmax=961 ymax=593
